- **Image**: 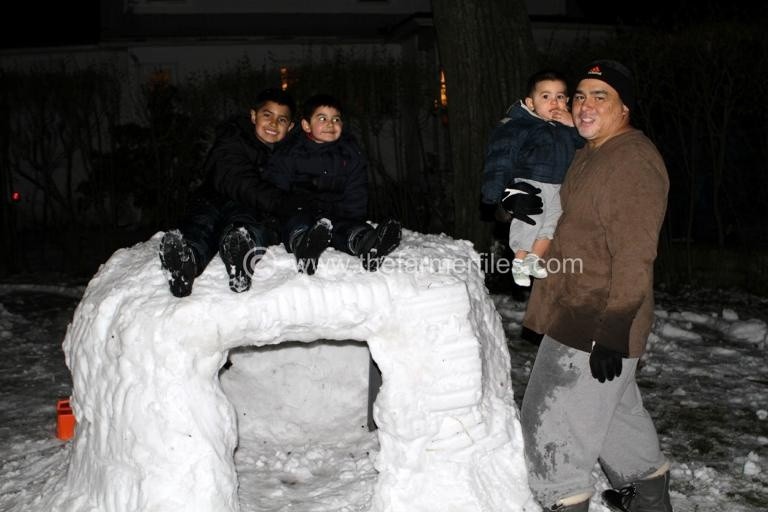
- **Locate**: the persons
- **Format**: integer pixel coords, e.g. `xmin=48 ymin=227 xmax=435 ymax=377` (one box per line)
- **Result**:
xmin=479 ymin=67 xmax=586 ymax=288
xmin=278 ymin=97 xmax=405 ymax=278
xmin=158 ymin=90 xmax=298 ymax=296
xmin=498 ymin=59 xmax=676 ymax=511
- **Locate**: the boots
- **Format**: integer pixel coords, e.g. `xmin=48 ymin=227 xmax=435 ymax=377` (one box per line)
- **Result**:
xmin=542 ymin=456 xmax=673 ymax=512
xmin=353 ymin=219 xmax=401 ymax=271
xmin=291 ymin=219 xmax=334 ymax=273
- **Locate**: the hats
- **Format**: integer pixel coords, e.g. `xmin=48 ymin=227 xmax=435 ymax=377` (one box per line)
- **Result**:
xmin=576 ymin=59 xmax=639 ymax=116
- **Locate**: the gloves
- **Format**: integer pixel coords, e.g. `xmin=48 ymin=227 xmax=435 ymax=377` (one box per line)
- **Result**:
xmin=502 ymin=181 xmax=543 ymax=224
xmin=590 ymin=346 xmax=623 ymax=381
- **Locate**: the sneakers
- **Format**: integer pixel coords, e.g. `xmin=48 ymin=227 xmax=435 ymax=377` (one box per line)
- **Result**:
xmin=159 ymin=232 xmax=195 ymax=296
xmin=219 ymin=228 xmax=253 ymax=294
